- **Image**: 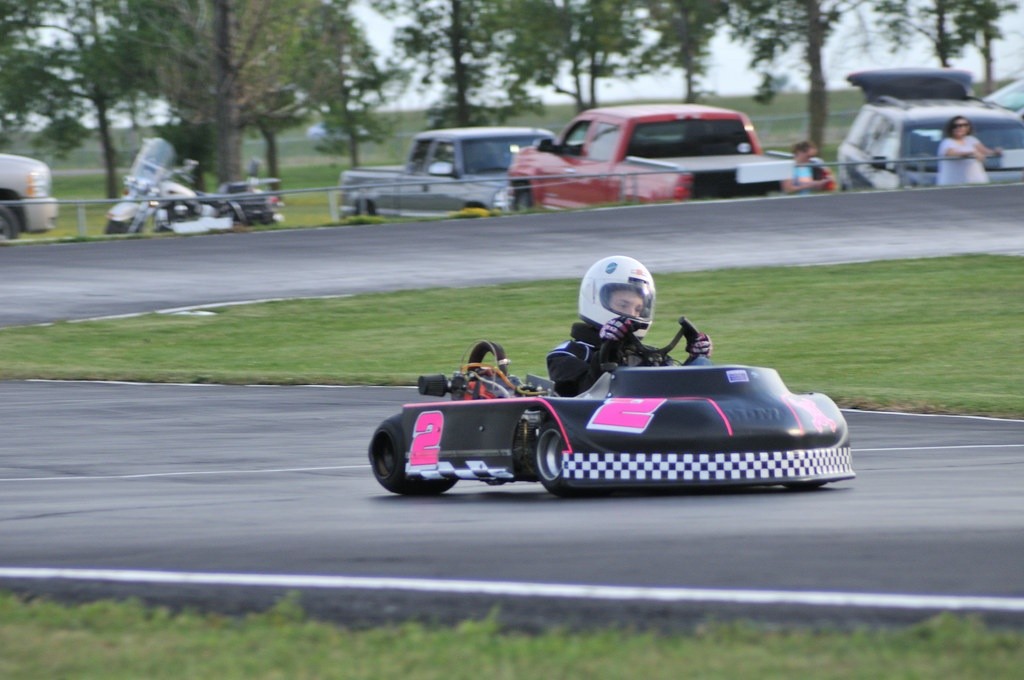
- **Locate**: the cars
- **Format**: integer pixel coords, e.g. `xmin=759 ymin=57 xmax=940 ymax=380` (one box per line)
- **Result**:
xmin=0 ymin=153 xmax=58 ymax=240
xmin=980 ymin=78 xmax=1023 ymax=114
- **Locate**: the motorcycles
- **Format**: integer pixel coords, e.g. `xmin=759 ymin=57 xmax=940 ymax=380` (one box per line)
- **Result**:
xmin=99 ymin=136 xmax=286 ymax=237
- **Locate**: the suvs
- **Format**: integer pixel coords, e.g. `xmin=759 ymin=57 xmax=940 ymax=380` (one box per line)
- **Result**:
xmin=835 ymin=68 xmax=1023 ymax=192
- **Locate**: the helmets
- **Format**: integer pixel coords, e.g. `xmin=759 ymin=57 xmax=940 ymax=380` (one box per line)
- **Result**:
xmin=578 ymin=255 xmax=655 ymax=340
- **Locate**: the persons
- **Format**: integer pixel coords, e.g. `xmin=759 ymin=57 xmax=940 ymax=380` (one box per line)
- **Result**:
xmin=935 ymin=116 xmax=1005 ymax=187
xmin=779 ymin=140 xmax=836 ymax=195
xmin=546 ymin=255 xmax=713 ymax=396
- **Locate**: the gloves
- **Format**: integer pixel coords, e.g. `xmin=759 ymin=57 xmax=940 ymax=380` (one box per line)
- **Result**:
xmin=685 ymin=332 xmax=711 ymax=361
xmin=599 ymin=316 xmax=632 ymax=347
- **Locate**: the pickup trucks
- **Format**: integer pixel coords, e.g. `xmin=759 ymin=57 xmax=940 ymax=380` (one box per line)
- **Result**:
xmin=334 ymin=127 xmax=553 ymax=219
xmin=504 ymin=104 xmax=836 ymax=208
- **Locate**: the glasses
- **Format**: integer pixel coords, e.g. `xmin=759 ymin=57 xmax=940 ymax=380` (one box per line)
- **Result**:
xmin=953 ymin=123 xmax=969 ymax=128
